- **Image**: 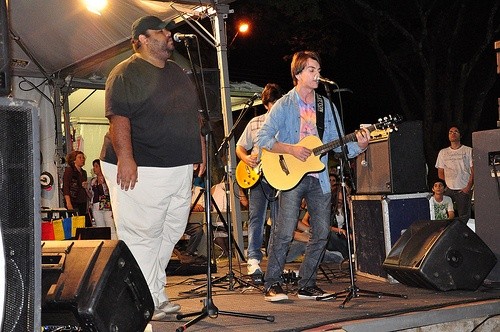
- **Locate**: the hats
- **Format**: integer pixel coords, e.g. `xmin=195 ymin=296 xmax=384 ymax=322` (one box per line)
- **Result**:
xmin=132 ymin=16 xmax=175 ymax=40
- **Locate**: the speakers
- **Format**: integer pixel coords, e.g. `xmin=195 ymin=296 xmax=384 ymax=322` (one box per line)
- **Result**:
xmin=164 ymin=238 xmax=217 ymax=276
xmin=354 ymin=120 xmax=427 ymax=195
xmin=0 ymin=96 xmax=42 ymax=332
xmin=472 ymin=129 xmax=500 ymax=283
xmin=381 ymin=218 xmax=497 ymax=292
xmin=40 ymin=240 xmax=155 ymax=332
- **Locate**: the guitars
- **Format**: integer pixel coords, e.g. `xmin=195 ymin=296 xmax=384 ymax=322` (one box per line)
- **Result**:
xmin=235 ymin=154 xmax=263 ymax=189
xmin=260 ymin=113 xmax=403 ymax=191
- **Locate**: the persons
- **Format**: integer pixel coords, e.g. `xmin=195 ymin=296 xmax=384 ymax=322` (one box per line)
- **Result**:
xmin=90 ymin=159 xmax=117 ymax=240
xmin=428 ymin=179 xmax=455 ymax=220
xmin=63 ymin=151 xmax=93 ymax=228
xmin=174 ymin=172 xmax=248 ymax=257
xmin=435 ymin=125 xmax=474 ymax=225
xmin=99 ymin=16 xmax=206 ymax=321
xmin=257 ymin=51 xmax=371 ymax=301
xmin=235 ymin=83 xmax=296 ymax=286
xmin=265 ymin=162 xmax=355 ymax=264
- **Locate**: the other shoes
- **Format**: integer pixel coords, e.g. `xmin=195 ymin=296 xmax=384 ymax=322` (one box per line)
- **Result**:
xmin=158 ymin=301 xmax=181 ymax=313
xmin=151 ymin=310 xmax=166 ymax=321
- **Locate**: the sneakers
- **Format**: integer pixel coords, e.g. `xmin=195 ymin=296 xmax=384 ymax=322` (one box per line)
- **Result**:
xmin=247 ymin=259 xmax=263 ymax=275
xmin=264 ymin=282 xmax=288 ymax=302
xmin=297 ymin=286 xmax=334 ymax=300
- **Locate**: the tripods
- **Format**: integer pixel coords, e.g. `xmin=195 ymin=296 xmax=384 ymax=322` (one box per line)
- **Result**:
xmin=176 ymin=41 xmax=275 ymax=332
xmin=191 ymin=102 xmax=266 ymax=294
xmin=316 ymin=85 xmax=408 ymax=310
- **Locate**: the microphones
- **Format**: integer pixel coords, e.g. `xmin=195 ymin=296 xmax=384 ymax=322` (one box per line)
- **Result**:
xmin=246 ymin=93 xmax=259 ymax=104
xmin=312 ymin=74 xmax=335 ymax=85
xmin=173 ymin=32 xmax=195 ymax=43
